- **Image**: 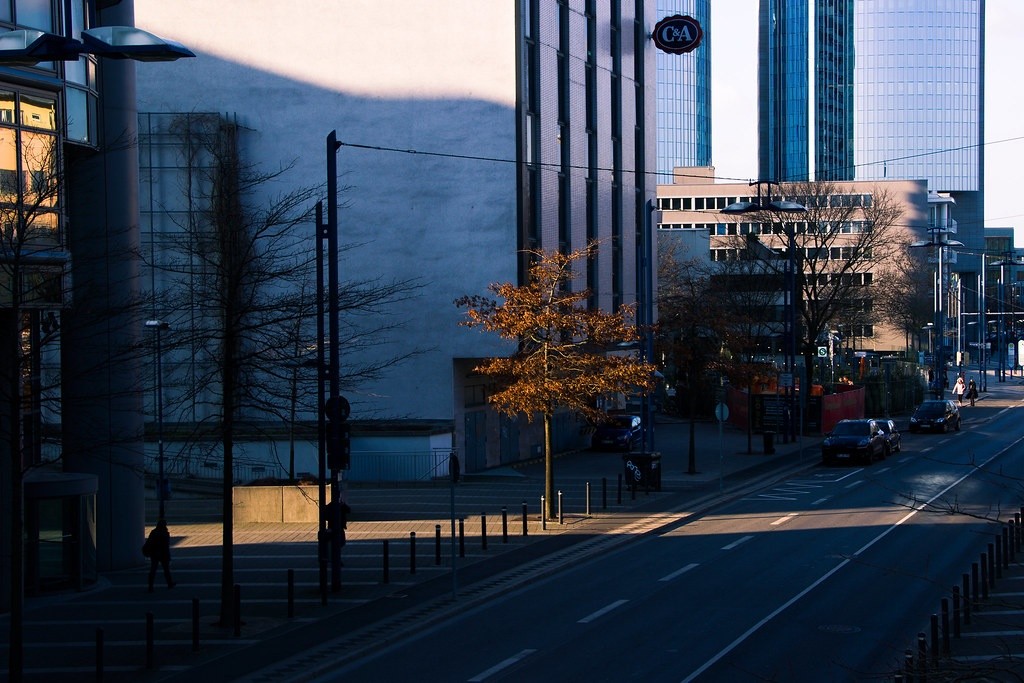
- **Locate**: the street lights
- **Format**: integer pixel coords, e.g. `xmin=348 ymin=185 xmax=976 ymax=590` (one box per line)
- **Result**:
xmin=145 ymin=319 xmax=172 ymax=522
xmin=967 ymin=319 xmax=987 ymax=391
xmin=987 ymin=318 xmax=1004 ymax=383
xmin=921 ymin=324 xmax=946 ymax=403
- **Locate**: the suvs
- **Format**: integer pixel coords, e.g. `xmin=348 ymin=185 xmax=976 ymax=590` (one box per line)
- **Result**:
xmin=821 ymin=416 xmax=886 ymax=464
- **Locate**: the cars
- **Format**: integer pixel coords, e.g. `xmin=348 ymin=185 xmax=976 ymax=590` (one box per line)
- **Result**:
xmin=592 ymin=414 xmax=645 ymax=453
xmin=909 ymin=400 xmax=962 ymax=433
xmin=874 ymin=416 xmax=901 ymax=455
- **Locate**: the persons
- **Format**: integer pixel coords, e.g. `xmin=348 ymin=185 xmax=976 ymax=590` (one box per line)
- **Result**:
xmin=965 ymin=378 xmax=979 ymax=406
xmin=42 ymin=311 xmax=60 ymax=335
xmin=145 ymin=520 xmax=178 ymax=595
xmin=950 ymin=377 xmax=966 ymax=405
